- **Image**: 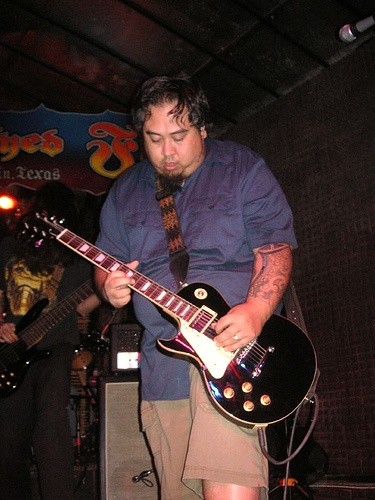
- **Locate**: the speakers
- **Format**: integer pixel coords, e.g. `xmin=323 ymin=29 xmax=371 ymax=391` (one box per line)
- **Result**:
xmin=96 ymin=375 xmax=161 ymax=500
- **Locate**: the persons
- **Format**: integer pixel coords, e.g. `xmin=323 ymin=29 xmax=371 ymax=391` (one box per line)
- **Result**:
xmin=0 ymin=182 xmax=102 ymax=500
xmin=93 ymin=75 xmax=300 ymax=500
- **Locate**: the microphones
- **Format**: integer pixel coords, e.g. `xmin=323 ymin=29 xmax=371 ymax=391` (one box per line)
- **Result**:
xmin=338 ymin=14 xmax=374 ymax=43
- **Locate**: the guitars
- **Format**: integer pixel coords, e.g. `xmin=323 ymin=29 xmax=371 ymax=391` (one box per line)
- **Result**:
xmin=0 ymin=267 xmax=96 ymax=395
xmin=17 ymin=202 xmax=320 ymax=432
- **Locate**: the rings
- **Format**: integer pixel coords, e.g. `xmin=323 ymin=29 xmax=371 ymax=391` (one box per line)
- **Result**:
xmin=232 ymin=336 xmax=240 ymax=340
